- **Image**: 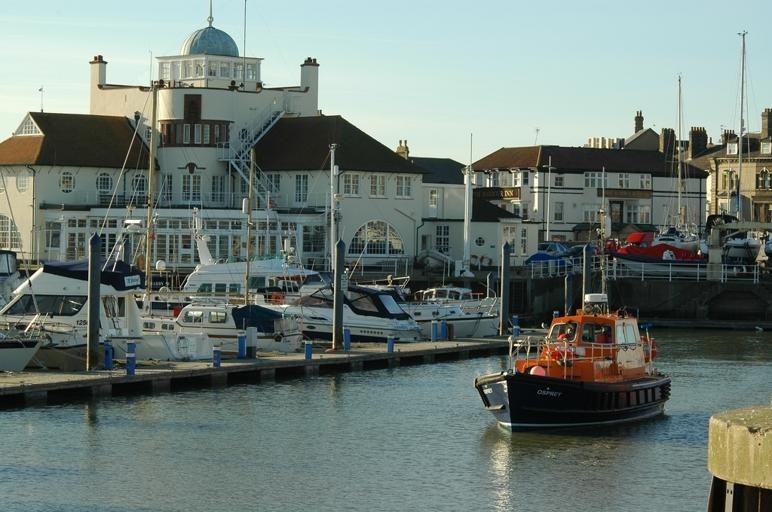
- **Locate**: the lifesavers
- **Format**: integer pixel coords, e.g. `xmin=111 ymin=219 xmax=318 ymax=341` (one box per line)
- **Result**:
xmin=640 ymin=336 xmax=656 ymax=362
xmin=272 ymin=292 xmax=284 ymax=304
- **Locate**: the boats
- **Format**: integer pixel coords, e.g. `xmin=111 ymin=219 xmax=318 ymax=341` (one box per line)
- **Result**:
xmin=476 ymin=208 xmax=668 ymax=432
